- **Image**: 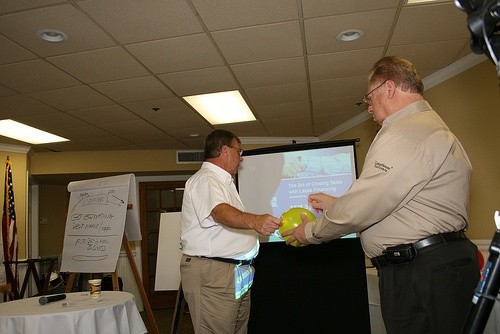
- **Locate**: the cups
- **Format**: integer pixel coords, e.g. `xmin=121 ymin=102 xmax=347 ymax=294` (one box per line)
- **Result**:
xmin=88 ymin=279 xmax=101 ymax=298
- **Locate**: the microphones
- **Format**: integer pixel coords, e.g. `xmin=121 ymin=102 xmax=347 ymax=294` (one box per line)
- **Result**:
xmin=39 ymin=294 xmax=66 ymax=305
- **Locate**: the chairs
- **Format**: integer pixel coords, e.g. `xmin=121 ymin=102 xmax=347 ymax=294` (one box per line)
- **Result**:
xmin=101 ymin=275 xmax=123 ymax=291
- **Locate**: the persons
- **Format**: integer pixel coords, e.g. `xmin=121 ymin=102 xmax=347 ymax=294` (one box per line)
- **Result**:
xmin=179 ymin=130 xmax=283 ymax=334
xmin=280 ymin=56 xmax=482 ymax=334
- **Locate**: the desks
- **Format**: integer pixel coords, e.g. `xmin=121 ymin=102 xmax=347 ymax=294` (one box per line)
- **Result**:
xmin=0 ymin=291 xmax=148 ymax=334
xmin=247 ymin=237 xmax=371 ymax=334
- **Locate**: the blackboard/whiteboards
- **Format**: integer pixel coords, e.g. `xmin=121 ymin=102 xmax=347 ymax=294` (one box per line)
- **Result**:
xmin=154 ymin=212 xmax=182 ymax=291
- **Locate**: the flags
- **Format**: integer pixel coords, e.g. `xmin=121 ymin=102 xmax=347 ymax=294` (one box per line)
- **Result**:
xmin=1 ymin=160 xmax=21 ymax=299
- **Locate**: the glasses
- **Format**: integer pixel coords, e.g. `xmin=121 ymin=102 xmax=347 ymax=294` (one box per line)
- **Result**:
xmin=227 ymin=144 xmax=244 ymax=156
xmin=362 ymin=80 xmax=388 ymax=106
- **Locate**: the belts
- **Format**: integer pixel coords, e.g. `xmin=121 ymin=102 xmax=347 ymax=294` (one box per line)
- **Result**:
xmin=202 ymin=256 xmax=253 ymax=265
xmin=370 ymin=232 xmax=457 ymax=268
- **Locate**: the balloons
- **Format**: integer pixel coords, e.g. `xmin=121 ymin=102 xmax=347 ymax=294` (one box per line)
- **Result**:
xmin=278 ymin=207 xmax=317 ymax=246
